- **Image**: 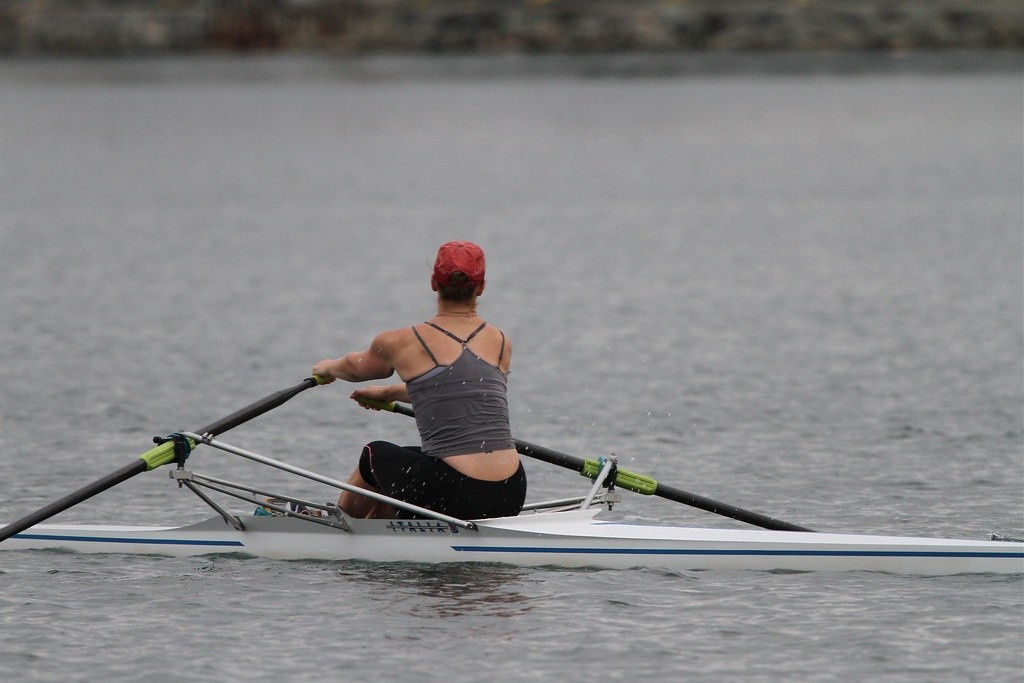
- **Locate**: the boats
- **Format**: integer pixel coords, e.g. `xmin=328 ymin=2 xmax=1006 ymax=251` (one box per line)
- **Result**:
xmin=0 ymin=506 xmax=1024 ymax=580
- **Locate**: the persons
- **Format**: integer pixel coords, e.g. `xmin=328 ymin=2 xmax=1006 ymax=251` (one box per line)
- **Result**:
xmin=290 ymin=240 xmax=528 ymax=520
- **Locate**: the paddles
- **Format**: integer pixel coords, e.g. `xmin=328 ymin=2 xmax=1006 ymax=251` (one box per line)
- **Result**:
xmin=366 ymin=398 xmax=818 ymax=533
xmin=0 ymin=372 xmax=331 ymax=542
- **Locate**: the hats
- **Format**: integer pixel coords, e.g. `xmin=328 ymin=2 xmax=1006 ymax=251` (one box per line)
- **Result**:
xmin=434 ymin=240 xmax=486 ymax=290
